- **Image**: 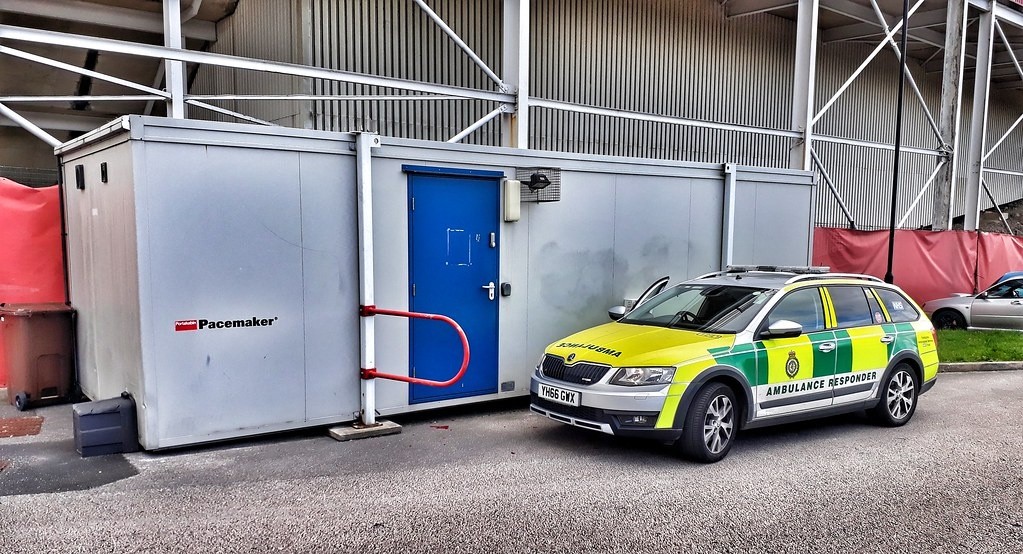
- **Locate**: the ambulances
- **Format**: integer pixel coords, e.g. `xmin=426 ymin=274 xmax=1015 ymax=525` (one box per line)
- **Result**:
xmin=530 ymin=265 xmax=940 ymax=463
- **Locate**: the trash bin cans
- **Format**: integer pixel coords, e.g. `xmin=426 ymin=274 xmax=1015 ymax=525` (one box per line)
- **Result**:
xmin=0 ymin=301 xmax=82 ymax=411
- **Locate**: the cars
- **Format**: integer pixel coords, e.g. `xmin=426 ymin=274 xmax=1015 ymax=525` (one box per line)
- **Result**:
xmin=919 ymin=272 xmax=1023 ymax=332
